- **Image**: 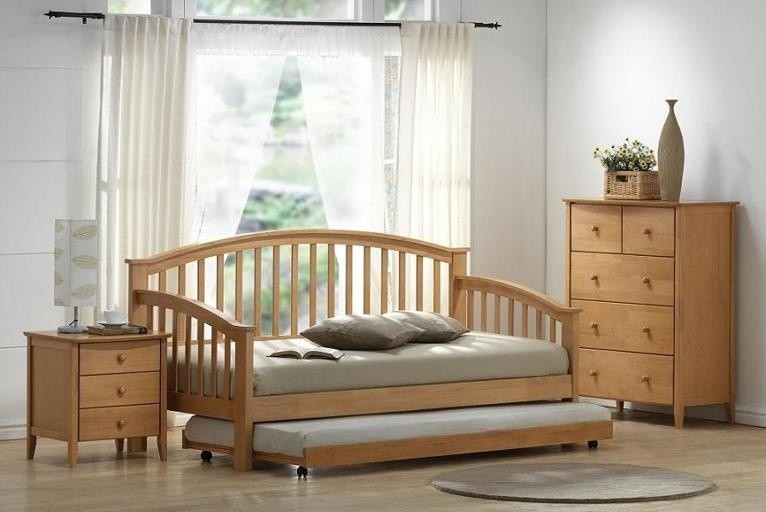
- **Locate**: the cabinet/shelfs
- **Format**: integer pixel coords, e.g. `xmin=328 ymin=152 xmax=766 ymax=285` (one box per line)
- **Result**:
xmin=21 ymin=330 xmax=172 ymax=469
xmin=560 ymin=192 xmax=740 ymax=429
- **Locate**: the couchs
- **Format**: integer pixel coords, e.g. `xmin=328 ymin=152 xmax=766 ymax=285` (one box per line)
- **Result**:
xmin=122 ymin=222 xmax=615 ymax=481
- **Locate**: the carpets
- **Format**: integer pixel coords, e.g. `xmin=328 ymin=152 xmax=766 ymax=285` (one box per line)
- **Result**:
xmin=425 ymin=458 xmax=717 ymax=505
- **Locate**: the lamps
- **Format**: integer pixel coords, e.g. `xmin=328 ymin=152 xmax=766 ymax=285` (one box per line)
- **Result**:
xmin=51 ymin=217 xmax=101 ymax=333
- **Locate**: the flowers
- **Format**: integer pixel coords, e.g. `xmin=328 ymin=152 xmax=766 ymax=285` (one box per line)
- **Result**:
xmin=592 ymin=135 xmax=657 ymax=183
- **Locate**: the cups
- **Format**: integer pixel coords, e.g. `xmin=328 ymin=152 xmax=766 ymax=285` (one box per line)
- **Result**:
xmin=104 ymin=311 xmax=128 ymax=324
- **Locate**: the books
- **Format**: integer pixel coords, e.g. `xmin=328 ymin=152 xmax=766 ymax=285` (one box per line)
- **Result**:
xmin=265 ymin=346 xmax=344 ymax=360
xmin=86 ymin=325 xmax=147 ymax=336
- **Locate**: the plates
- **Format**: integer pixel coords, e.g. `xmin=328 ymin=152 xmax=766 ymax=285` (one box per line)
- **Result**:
xmin=98 ymin=322 xmax=130 ymax=327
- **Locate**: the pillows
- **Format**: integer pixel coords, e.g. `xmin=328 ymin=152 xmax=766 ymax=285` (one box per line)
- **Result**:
xmin=294 ymin=309 xmax=471 ymax=349
